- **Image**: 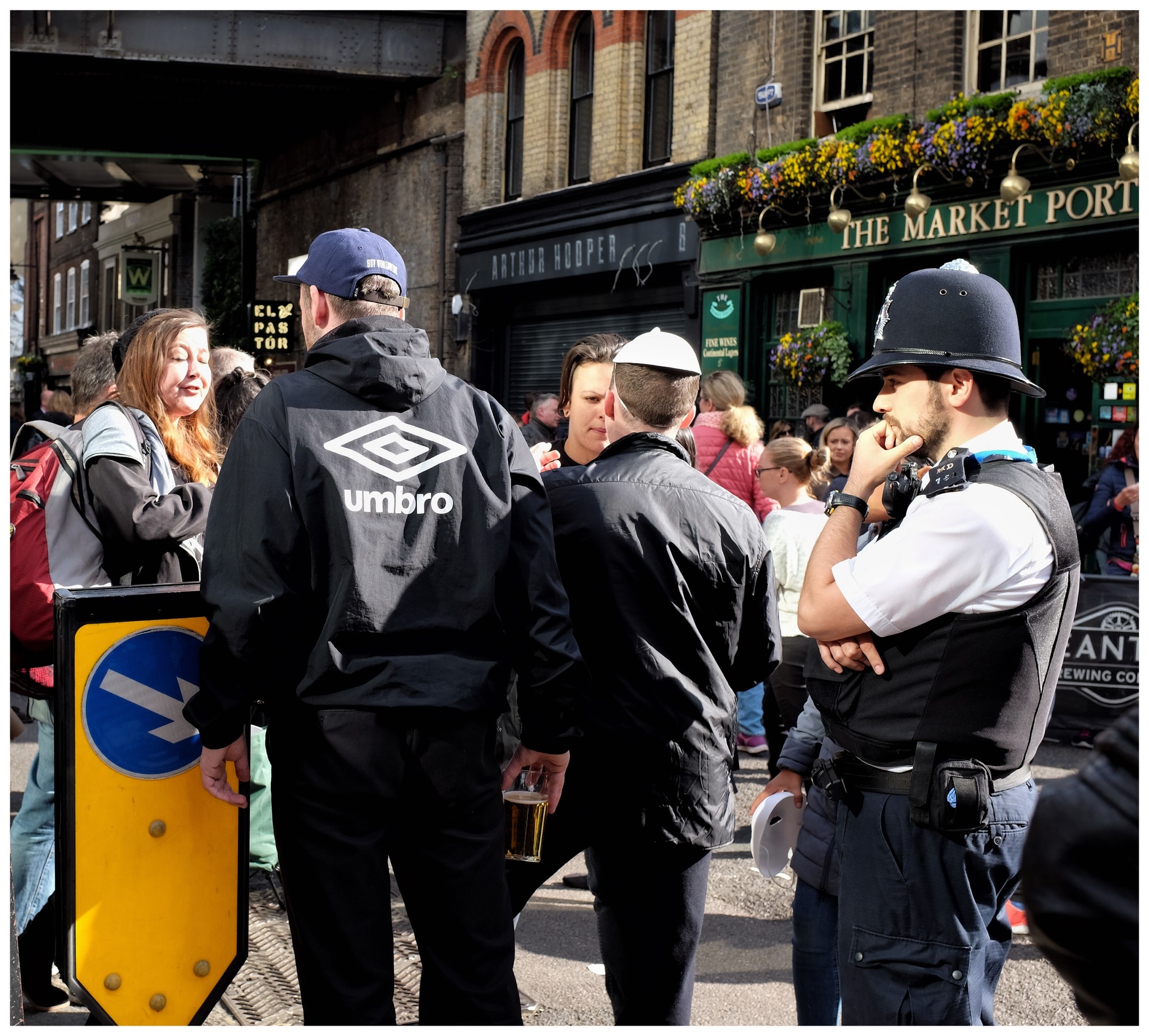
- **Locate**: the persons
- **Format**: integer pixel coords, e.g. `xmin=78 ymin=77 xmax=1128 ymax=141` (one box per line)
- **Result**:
xmin=749 ymin=443 xmax=933 ymax=1025
xmin=1019 ymin=705 xmax=1139 ymax=1026
xmin=798 ymin=270 xmax=1080 ymax=1026
xmin=198 ymin=228 xmax=594 ymax=1027
xmin=1079 ymin=419 xmax=1139 ymax=578
xmin=11 ymin=332 xmax=1031 ymax=934
xmin=10 ymin=310 xmax=220 ymax=1014
xmin=495 ymin=327 xmax=784 ymax=1026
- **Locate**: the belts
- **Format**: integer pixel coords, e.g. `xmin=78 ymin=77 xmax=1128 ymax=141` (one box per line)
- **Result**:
xmin=830 ymin=751 xmax=1030 ymax=797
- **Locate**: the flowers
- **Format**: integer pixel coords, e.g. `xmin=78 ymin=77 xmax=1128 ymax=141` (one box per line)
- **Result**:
xmin=767 ymin=319 xmax=854 ymax=387
xmin=14 ymin=352 xmax=42 ymax=369
xmin=676 ymin=65 xmax=1139 ymax=219
xmin=1061 ymin=290 xmax=1139 ymax=380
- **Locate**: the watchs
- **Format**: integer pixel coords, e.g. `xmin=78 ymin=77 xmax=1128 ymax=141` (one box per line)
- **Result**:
xmin=823 ymin=489 xmax=869 ymax=522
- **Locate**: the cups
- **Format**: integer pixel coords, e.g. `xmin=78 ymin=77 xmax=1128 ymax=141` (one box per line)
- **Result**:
xmin=503 ymin=769 xmax=550 ymax=860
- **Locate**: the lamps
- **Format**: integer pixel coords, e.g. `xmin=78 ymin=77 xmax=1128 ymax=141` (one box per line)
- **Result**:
xmin=827 ymin=182 xmax=886 ymax=234
xmin=10 ymin=263 xmax=37 ymax=284
xmin=1000 ymin=143 xmax=1076 ymax=202
xmin=904 ymin=164 xmax=974 ymax=218
xmin=1118 ymin=121 xmax=1139 ymax=184
xmin=754 ymin=205 xmax=810 ymax=257
xmin=451 ymin=294 xmax=471 ymax=343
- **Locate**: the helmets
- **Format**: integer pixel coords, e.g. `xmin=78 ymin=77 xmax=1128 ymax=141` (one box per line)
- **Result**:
xmin=846 ymin=259 xmax=1046 ymax=399
xmin=613 ymin=325 xmax=701 ymax=373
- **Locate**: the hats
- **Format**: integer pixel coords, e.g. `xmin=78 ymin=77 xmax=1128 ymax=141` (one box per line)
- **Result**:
xmin=273 ymin=227 xmax=410 ymax=308
xmin=800 ymin=403 xmax=829 ymax=418
xmin=111 ymin=308 xmax=176 ymax=373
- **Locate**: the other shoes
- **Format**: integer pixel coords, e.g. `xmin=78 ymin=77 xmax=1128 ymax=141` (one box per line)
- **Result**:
xmin=736 ymin=732 xmax=768 ymax=754
xmin=1071 ymin=728 xmax=1095 ymax=749
xmin=1004 ymin=899 xmax=1031 ymax=935
xmin=1043 ymin=722 xmax=1070 ymax=743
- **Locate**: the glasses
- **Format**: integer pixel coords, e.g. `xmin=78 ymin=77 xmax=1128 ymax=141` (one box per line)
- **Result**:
xmin=779 ymin=428 xmax=792 ymax=436
xmin=754 ymin=467 xmax=793 ymax=478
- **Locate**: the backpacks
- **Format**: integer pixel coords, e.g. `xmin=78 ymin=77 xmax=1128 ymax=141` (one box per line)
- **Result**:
xmin=10 ymin=400 xmax=152 ymax=701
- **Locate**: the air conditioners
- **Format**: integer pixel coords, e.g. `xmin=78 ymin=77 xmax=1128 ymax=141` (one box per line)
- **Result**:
xmin=797 ymin=289 xmax=825 ymax=328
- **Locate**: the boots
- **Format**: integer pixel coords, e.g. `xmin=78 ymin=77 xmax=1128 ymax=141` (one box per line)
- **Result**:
xmin=17 ymin=919 xmax=71 ymax=1010
